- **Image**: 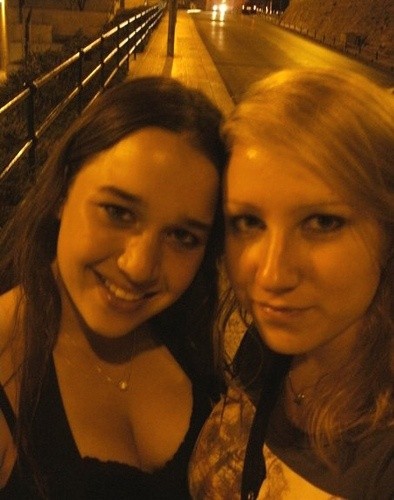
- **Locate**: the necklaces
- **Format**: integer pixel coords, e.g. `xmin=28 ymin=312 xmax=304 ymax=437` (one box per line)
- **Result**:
xmin=288 ymin=377 xmax=306 ymax=404
xmin=55 ymin=325 xmax=137 ymax=390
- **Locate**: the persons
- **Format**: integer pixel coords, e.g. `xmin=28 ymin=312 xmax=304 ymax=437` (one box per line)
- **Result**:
xmin=187 ymin=68 xmax=394 ymax=500
xmin=0 ymin=76 xmax=225 ymax=500
